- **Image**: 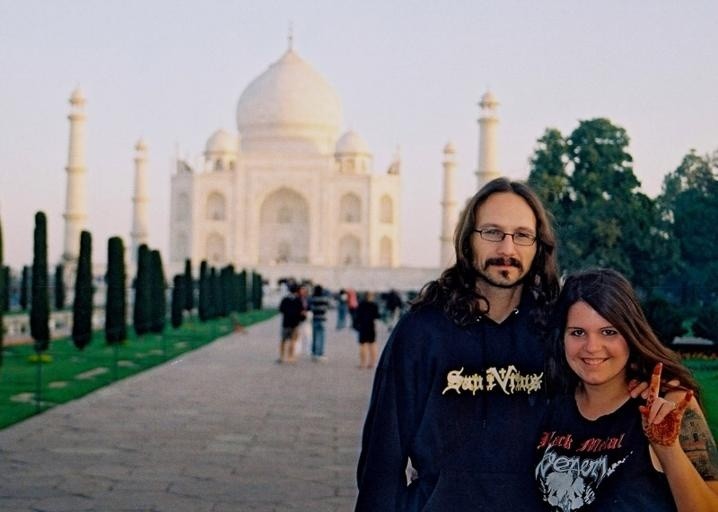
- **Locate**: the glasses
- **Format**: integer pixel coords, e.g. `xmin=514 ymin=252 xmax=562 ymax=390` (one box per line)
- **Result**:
xmin=473 ymin=228 xmax=538 ymax=246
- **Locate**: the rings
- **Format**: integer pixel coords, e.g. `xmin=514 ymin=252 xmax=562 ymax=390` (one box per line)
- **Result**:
xmin=669 ymin=400 xmax=677 ymax=409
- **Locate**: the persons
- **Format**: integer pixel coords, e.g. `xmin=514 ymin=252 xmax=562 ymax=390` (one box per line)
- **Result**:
xmin=259 ymin=276 xmax=406 ymax=372
xmin=353 ymin=175 xmax=687 ymax=511
xmin=530 ymin=263 xmax=718 ymax=512
